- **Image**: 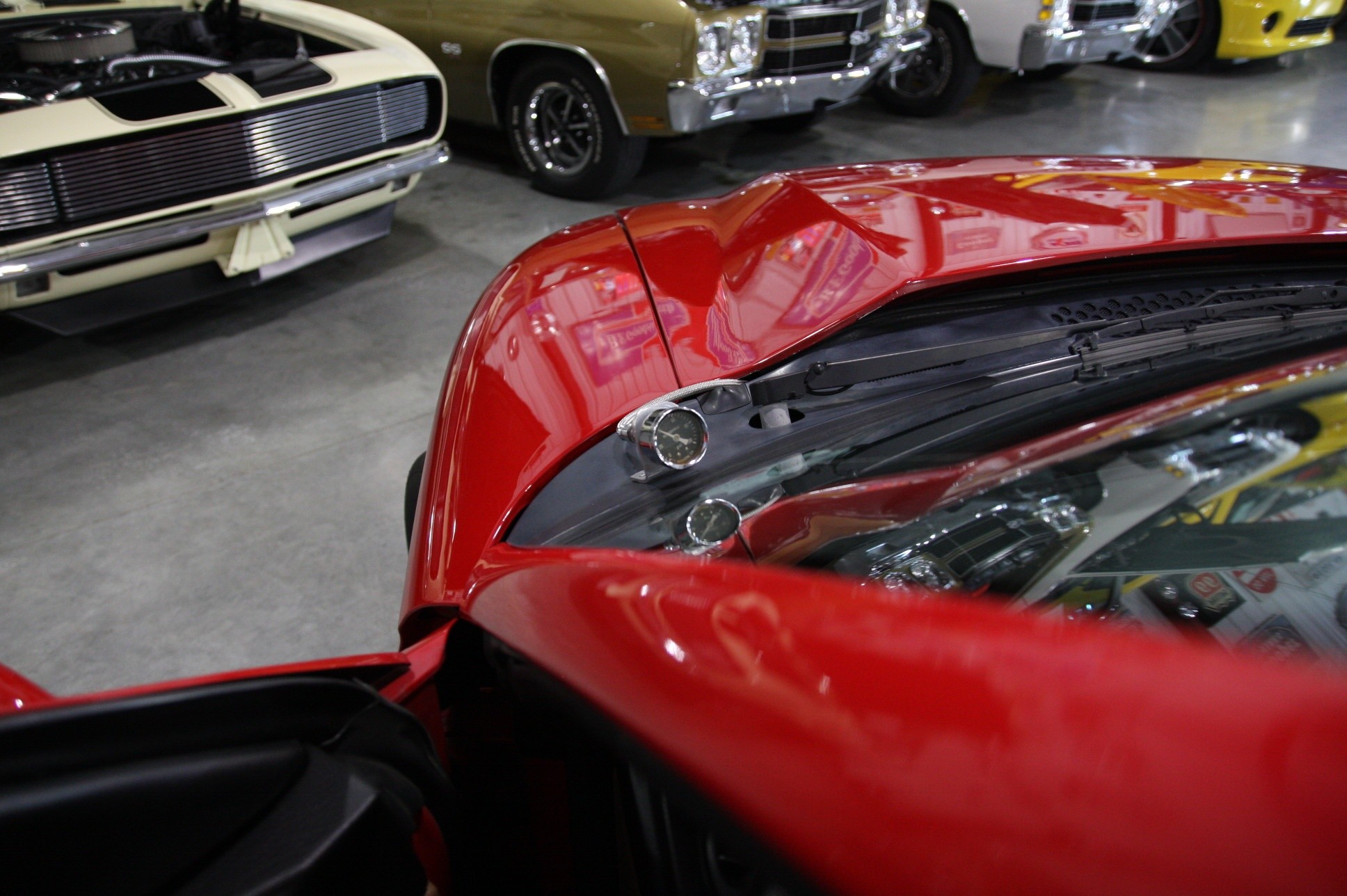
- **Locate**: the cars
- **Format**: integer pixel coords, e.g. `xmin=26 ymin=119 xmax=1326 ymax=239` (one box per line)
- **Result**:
xmin=0 ymin=150 xmax=1347 ymax=896
xmin=304 ymin=0 xmax=938 ymax=199
xmin=1120 ymin=0 xmax=1346 ymax=72
xmin=873 ymin=0 xmax=1179 ymax=116
xmin=0 ymin=0 xmax=455 ymax=343
